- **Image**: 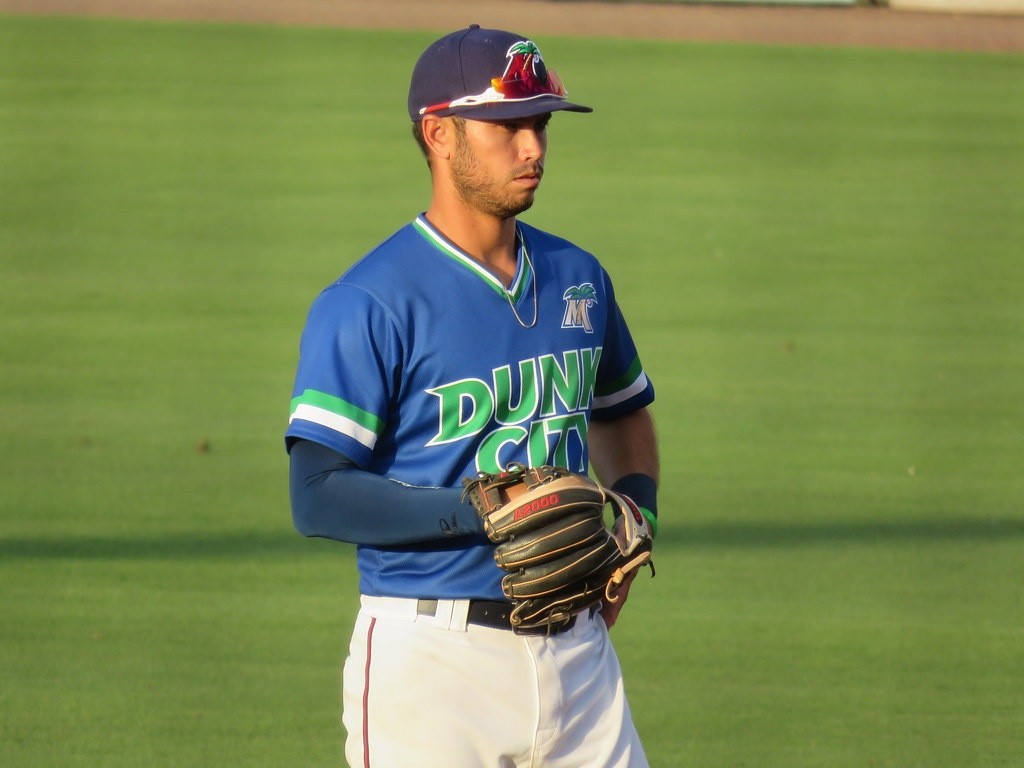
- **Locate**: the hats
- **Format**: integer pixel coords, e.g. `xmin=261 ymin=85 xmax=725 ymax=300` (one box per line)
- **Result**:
xmin=406 ymin=26 xmax=593 ymax=121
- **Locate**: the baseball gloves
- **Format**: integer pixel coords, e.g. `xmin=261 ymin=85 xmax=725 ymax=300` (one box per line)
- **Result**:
xmin=466 ymin=464 xmax=655 ymax=638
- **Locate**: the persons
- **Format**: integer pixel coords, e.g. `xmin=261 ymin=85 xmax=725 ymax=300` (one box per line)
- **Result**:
xmin=286 ymin=26 xmax=659 ymax=768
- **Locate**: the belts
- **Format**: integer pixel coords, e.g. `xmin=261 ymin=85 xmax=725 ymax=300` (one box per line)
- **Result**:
xmin=414 ymin=596 xmax=578 ymax=634
